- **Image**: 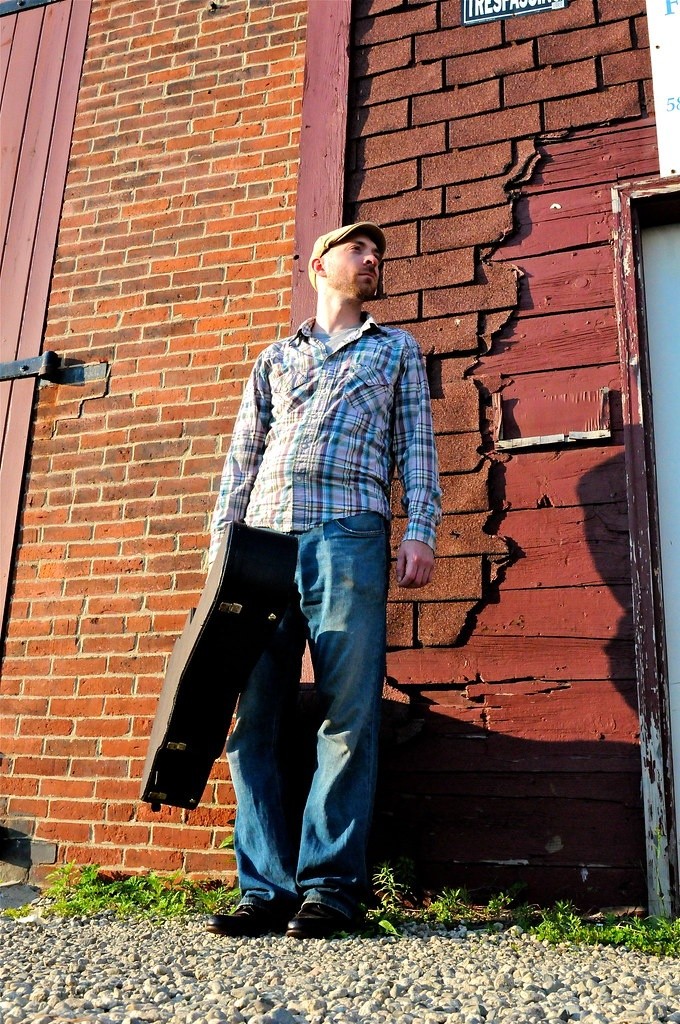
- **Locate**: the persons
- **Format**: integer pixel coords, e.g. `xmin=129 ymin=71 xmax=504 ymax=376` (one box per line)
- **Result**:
xmin=206 ymin=221 xmax=443 ymax=938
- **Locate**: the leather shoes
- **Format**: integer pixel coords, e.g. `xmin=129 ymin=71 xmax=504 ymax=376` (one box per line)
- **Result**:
xmin=285 ymin=903 xmax=348 ymax=938
xmin=205 ymin=905 xmax=271 ymax=935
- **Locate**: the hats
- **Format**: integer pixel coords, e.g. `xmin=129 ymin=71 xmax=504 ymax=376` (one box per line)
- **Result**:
xmin=308 ymin=222 xmax=387 ymax=292
